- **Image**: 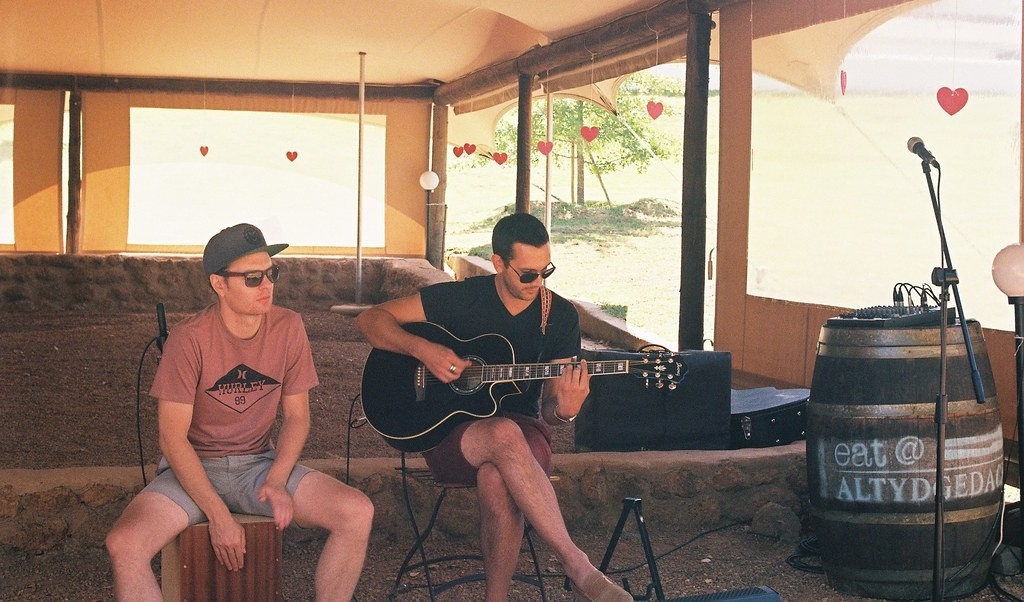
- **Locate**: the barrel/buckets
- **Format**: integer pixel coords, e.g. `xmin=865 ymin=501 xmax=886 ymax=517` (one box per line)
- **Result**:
xmin=806 ymin=319 xmax=1003 ymax=599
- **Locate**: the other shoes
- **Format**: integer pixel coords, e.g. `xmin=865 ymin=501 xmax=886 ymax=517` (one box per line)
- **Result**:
xmin=570 ymin=568 xmax=633 ymax=602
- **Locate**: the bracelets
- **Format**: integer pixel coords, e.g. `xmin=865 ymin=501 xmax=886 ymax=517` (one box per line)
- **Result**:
xmin=554 ymin=405 xmax=577 ymax=423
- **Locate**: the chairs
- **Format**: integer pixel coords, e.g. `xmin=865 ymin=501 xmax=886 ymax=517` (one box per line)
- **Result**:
xmin=384 ymin=448 xmax=548 ymax=602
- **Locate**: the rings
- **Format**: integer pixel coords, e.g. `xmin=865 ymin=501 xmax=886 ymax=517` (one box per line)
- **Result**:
xmin=450 ymin=365 xmax=456 ymax=372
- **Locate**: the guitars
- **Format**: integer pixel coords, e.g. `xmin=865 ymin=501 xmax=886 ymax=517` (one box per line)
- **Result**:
xmin=360 ymin=321 xmax=692 ymax=455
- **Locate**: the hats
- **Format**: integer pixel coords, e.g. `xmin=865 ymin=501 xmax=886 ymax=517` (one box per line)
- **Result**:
xmin=203 ymin=224 xmax=288 ymax=281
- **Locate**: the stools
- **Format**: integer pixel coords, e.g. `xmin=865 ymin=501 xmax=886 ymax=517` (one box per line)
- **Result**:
xmin=160 ymin=511 xmax=279 ymax=602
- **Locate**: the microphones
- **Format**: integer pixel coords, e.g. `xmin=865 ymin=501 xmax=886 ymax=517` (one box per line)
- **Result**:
xmin=907 ymin=137 xmax=940 ymax=168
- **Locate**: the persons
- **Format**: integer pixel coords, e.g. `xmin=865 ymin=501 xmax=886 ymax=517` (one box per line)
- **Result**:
xmin=356 ymin=212 xmax=633 ymax=602
xmin=105 ymin=223 xmax=374 ymax=602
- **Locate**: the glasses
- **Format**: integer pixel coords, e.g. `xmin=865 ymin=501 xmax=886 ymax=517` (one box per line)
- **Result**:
xmin=497 ymin=251 xmax=556 ymax=283
xmin=217 ymin=264 xmax=280 ymax=287
